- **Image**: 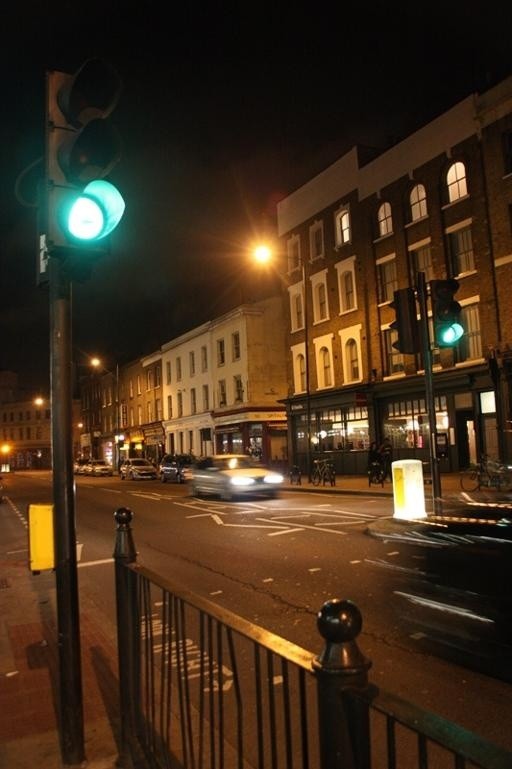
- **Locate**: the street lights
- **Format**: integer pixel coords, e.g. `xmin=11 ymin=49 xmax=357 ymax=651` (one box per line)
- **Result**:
xmin=92 ymin=358 xmax=121 ymax=474
xmin=255 ymin=245 xmax=314 ymax=482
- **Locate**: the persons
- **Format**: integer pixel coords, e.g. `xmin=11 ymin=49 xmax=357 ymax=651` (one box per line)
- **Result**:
xmin=337 ymin=442 xmax=343 ymax=450
xmin=245 ymin=443 xmax=264 ymax=457
xmin=147 ymin=452 xmax=167 ymax=468
xmin=118 ymin=456 xmax=124 ymax=469
xmin=367 ymin=437 xmax=393 ymax=483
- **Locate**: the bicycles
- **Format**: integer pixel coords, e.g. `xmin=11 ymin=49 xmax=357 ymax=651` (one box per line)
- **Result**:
xmin=460 ymin=455 xmax=512 ymax=493
xmin=312 ymin=459 xmax=336 ymax=486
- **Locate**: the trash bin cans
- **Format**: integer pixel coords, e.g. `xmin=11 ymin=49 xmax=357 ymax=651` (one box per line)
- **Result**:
xmin=29 ymin=502 xmax=54 ymax=574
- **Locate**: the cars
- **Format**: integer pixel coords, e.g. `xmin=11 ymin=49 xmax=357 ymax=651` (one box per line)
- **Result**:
xmin=120 ymin=458 xmax=156 ymax=480
xmin=191 ymin=454 xmax=283 ymax=501
xmin=73 ymin=458 xmax=114 ymax=477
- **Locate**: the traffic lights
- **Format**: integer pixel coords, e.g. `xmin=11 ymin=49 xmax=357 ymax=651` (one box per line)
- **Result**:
xmin=429 ymin=278 xmax=464 ymax=348
xmin=45 ymin=59 xmax=125 ymax=262
xmin=388 ymin=287 xmax=420 ymax=355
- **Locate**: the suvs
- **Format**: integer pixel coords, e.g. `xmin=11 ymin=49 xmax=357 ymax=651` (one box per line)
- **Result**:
xmin=159 ymin=454 xmax=199 ymax=484
